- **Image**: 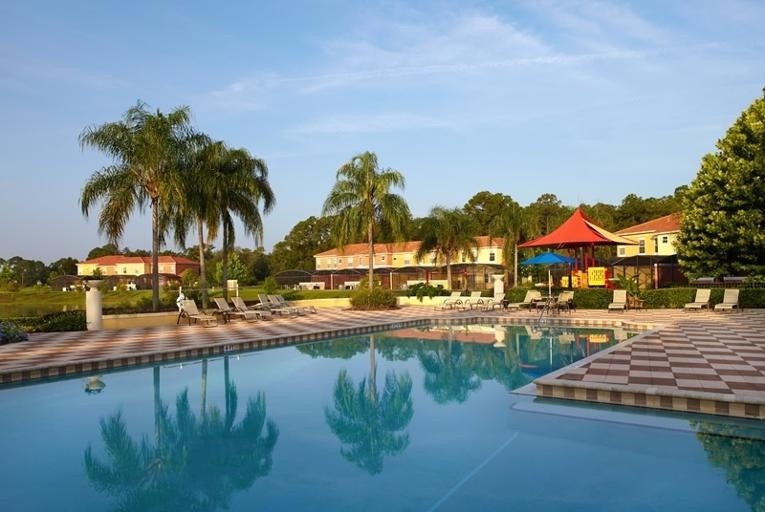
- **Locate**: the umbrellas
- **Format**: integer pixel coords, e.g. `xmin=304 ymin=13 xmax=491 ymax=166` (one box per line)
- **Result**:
xmin=519 ymin=250 xmax=577 ymax=294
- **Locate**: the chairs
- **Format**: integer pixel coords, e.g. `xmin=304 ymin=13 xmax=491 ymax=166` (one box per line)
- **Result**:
xmin=433 ymin=288 xmax=577 ymax=317
xmin=607 ymin=288 xmax=630 ymax=311
xmin=176 ymin=293 xmax=316 ymax=328
xmin=683 ymin=287 xmax=742 ymax=312
